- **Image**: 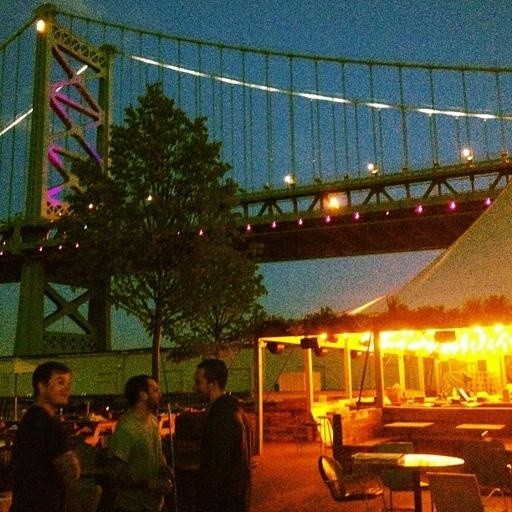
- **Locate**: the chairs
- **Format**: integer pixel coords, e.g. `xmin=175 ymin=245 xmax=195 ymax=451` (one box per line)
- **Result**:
xmin=318 ymin=456 xmax=508 ymax=511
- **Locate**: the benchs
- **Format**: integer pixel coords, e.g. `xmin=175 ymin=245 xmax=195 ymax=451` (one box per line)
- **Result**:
xmin=333 ymin=407 xmax=511 ymax=454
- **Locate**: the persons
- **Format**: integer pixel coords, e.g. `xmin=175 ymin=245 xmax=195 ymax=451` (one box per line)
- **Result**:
xmin=194 ymin=358 xmax=254 ymax=512
xmin=101 ymin=374 xmax=176 ymax=512
xmin=9 ymin=359 xmax=84 ymax=511
